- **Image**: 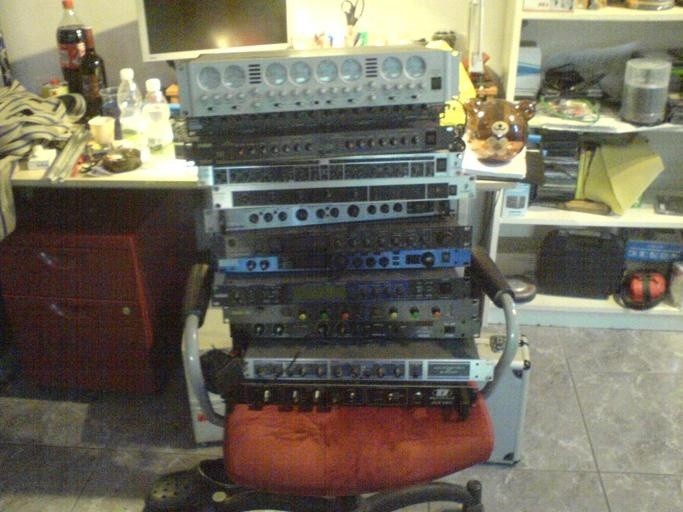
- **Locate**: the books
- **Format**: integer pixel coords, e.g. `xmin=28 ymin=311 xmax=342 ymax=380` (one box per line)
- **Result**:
xmin=527 ymin=129 xmax=613 ymax=216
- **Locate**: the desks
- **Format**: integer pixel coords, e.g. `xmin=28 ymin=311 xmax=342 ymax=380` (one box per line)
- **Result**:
xmin=0 ymin=97 xmax=528 ymax=237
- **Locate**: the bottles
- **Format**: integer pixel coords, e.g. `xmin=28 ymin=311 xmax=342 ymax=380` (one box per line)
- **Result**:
xmin=42 ymin=1 xmax=107 ymax=116
xmin=118 ymin=68 xmax=176 ymax=165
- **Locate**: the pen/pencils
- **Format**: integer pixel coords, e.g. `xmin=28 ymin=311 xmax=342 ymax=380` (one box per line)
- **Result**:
xmin=352 ymin=31 xmax=368 ymax=49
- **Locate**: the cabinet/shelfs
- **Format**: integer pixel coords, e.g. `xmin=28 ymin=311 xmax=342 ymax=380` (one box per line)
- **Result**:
xmin=481 ymin=0 xmax=683 ymax=331
xmin=0 ymin=191 xmax=196 ymax=394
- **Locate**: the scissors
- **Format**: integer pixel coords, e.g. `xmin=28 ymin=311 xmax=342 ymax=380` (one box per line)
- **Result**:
xmin=340 ymin=0 xmax=364 ymax=37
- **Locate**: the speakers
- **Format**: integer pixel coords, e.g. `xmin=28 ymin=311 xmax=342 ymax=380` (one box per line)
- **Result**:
xmin=537 ymin=228 xmax=627 ymax=298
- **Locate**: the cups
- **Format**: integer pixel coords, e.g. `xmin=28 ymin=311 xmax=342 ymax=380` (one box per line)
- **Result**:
xmin=100 ymin=88 xmax=120 ymax=120
xmin=88 ymin=118 xmax=114 ymax=146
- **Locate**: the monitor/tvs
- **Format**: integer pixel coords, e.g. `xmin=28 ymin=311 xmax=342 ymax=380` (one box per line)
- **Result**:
xmin=134 ymin=0 xmax=289 ymax=65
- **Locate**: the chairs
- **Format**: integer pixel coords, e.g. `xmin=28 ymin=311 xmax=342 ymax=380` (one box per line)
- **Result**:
xmin=143 ymin=230 xmax=518 ymax=512
